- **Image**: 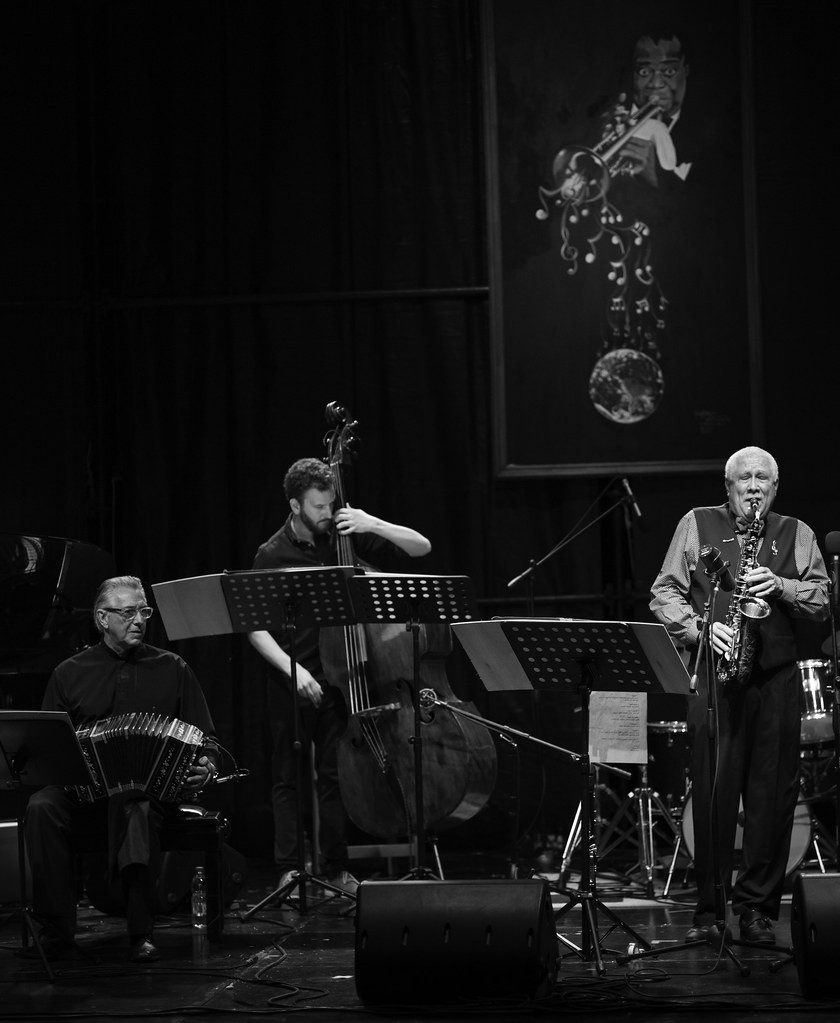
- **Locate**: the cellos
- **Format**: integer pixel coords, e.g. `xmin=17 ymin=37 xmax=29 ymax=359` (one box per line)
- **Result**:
xmin=322 ymin=400 xmax=500 ymax=880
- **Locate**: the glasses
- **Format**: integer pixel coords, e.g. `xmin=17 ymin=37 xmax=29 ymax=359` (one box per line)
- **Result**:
xmin=103 ymin=607 xmax=153 ymax=621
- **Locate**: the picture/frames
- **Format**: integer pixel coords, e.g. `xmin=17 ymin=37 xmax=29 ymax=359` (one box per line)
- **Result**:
xmin=483 ymin=0 xmax=765 ymax=478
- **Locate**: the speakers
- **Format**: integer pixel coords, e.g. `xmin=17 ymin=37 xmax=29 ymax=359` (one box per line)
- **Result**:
xmin=792 ymin=872 xmax=840 ymax=1003
xmin=87 ymin=848 xmax=205 ymax=915
xmin=355 ymin=876 xmax=560 ymax=1010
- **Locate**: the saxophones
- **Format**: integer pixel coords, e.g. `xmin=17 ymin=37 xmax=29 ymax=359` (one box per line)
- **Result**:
xmin=716 ymin=497 xmax=773 ymax=684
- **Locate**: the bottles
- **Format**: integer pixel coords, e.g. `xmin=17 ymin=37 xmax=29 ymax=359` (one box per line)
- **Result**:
xmin=191 ymin=867 xmax=209 ymax=928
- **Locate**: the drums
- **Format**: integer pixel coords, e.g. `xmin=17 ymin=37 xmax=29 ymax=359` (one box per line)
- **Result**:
xmin=680 ymin=777 xmax=813 ymax=886
xmin=628 ymin=719 xmax=687 ymax=818
xmin=796 ymin=659 xmax=836 ymax=745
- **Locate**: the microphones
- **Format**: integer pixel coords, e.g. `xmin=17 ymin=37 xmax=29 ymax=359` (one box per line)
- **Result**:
xmin=622 ymin=478 xmax=642 ymax=517
xmin=698 ymin=544 xmax=736 ymax=592
xmin=825 ymin=531 xmax=840 ymax=608
xmin=418 ymin=689 xmax=438 ymax=708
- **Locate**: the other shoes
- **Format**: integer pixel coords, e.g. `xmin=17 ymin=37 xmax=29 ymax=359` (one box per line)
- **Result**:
xmin=126 ymin=935 xmax=162 ymax=962
xmin=39 ymin=930 xmax=81 ymax=961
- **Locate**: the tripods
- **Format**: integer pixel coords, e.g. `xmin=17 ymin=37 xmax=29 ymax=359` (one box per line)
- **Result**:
xmin=219 ymin=565 xmax=792 ymax=975
xmin=0 ymin=709 xmax=96 ymax=983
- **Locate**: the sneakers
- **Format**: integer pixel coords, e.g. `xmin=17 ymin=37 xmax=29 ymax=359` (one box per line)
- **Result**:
xmin=686 ymin=926 xmax=709 ymax=942
xmin=324 ymin=870 xmax=365 ymax=899
xmin=277 ymin=869 xmax=300 ymax=899
xmin=739 ymin=910 xmax=777 ymax=941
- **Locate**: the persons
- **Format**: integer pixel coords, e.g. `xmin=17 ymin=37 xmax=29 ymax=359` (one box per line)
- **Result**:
xmin=648 ymin=447 xmax=832 ymax=946
xmin=598 ymin=33 xmax=698 ymax=182
xmin=14 ymin=576 xmax=218 ymax=967
xmin=241 ymin=457 xmax=432 ymax=897
